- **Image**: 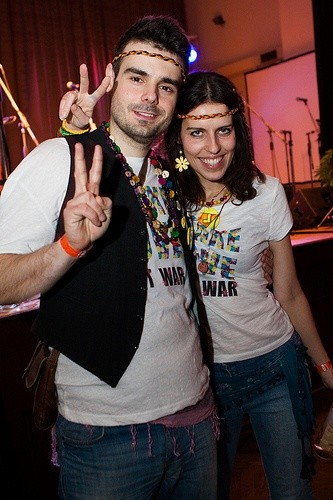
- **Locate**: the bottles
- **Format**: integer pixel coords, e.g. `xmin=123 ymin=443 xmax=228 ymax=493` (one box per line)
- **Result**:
xmin=312 ymin=404 xmax=333 ymax=460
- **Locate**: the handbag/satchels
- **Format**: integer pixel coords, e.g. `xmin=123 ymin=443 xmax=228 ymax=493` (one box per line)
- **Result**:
xmin=22 ymin=341 xmax=60 ymax=429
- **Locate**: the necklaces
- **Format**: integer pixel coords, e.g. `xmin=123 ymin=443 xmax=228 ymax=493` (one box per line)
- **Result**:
xmin=101 ymin=121 xmax=181 ymax=245
xmin=184 ymin=180 xmax=237 ymax=273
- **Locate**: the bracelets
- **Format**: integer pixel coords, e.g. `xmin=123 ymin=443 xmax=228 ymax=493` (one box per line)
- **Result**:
xmin=313 ymin=361 xmax=332 ymax=373
xmin=59 ymin=234 xmax=93 ymax=259
xmin=56 ymin=119 xmax=90 ymax=137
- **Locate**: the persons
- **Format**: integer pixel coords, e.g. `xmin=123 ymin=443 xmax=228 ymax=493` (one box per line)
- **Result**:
xmin=59 ymin=63 xmax=333 ymax=500
xmin=0 ymin=15 xmax=274 ymax=500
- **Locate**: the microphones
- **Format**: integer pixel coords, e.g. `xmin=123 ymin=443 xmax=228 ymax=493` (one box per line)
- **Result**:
xmin=296 ymin=97 xmax=307 ymax=102
xmin=306 ymin=131 xmax=315 ymax=135
